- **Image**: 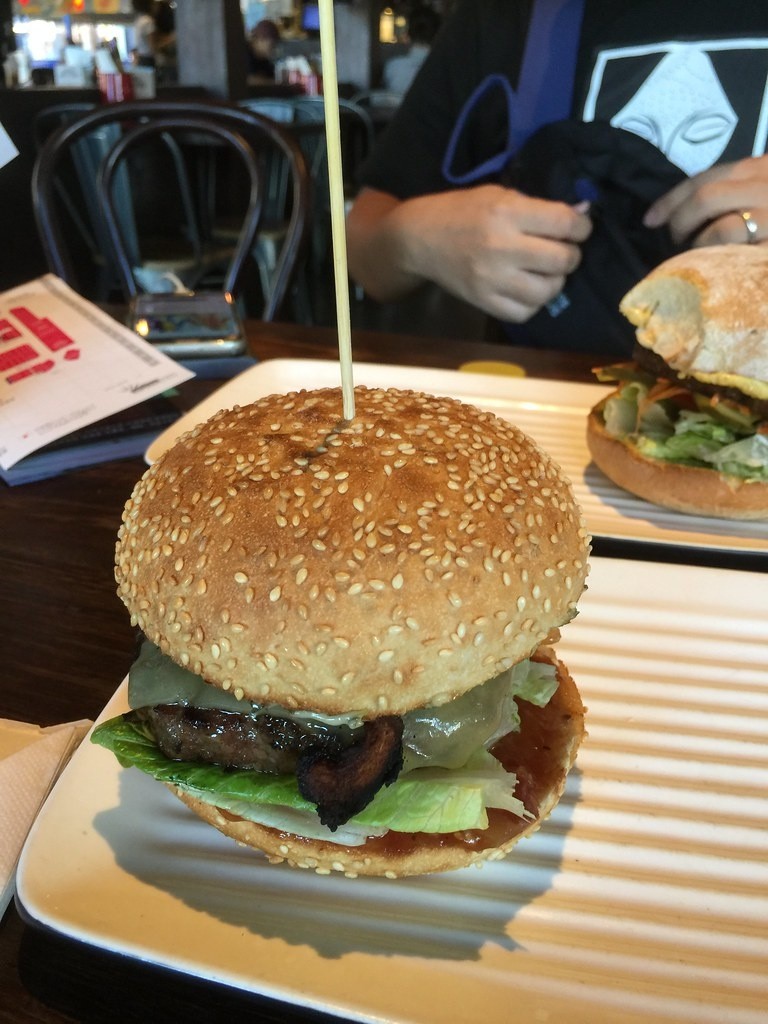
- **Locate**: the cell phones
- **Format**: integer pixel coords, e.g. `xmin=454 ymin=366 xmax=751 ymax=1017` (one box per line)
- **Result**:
xmin=126 ymin=289 xmax=247 ymax=359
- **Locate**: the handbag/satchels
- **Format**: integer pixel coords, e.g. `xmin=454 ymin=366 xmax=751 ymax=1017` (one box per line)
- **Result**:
xmin=447 ymin=121 xmax=712 ymax=348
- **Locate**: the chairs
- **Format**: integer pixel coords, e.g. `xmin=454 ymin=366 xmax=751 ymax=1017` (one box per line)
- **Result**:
xmin=30 ymin=89 xmax=406 ymax=322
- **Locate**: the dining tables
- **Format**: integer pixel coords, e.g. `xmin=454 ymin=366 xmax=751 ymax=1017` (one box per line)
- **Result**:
xmin=0 ymin=302 xmax=768 ymax=1023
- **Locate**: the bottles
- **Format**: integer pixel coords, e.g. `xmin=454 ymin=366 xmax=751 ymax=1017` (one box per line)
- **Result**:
xmin=378 ymin=9 xmax=413 ymax=45
xmin=275 ymin=54 xmax=322 ymax=100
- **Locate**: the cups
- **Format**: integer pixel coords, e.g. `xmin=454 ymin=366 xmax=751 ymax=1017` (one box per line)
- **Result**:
xmin=94 ymin=47 xmax=118 ymax=75
xmin=129 ymin=67 xmax=157 ymax=101
xmin=96 ymin=72 xmax=124 ymax=103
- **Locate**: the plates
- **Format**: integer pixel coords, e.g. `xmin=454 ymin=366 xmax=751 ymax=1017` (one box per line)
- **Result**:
xmin=145 ymin=358 xmax=768 ymax=555
xmin=14 ymin=551 xmax=768 ymax=1024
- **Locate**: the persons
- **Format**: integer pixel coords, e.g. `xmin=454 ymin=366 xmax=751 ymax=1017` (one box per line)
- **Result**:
xmin=344 ymin=0 xmax=768 ymax=360
xmin=383 ymin=9 xmax=442 ymax=92
xmin=131 ymin=0 xmax=173 ymax=68
xmin=243 ymin=20 xmax=289 ymax=84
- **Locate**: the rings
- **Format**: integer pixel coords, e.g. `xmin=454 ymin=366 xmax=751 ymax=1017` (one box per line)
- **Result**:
xmin=735 ymin=209 xmax=757 ymax=243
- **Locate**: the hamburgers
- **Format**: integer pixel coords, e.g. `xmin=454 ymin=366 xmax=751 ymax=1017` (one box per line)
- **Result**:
xmin=587 ymin=244 xmax=768 ymax=522
xmin=89 ymin=387 xmax=592 ymax=877
